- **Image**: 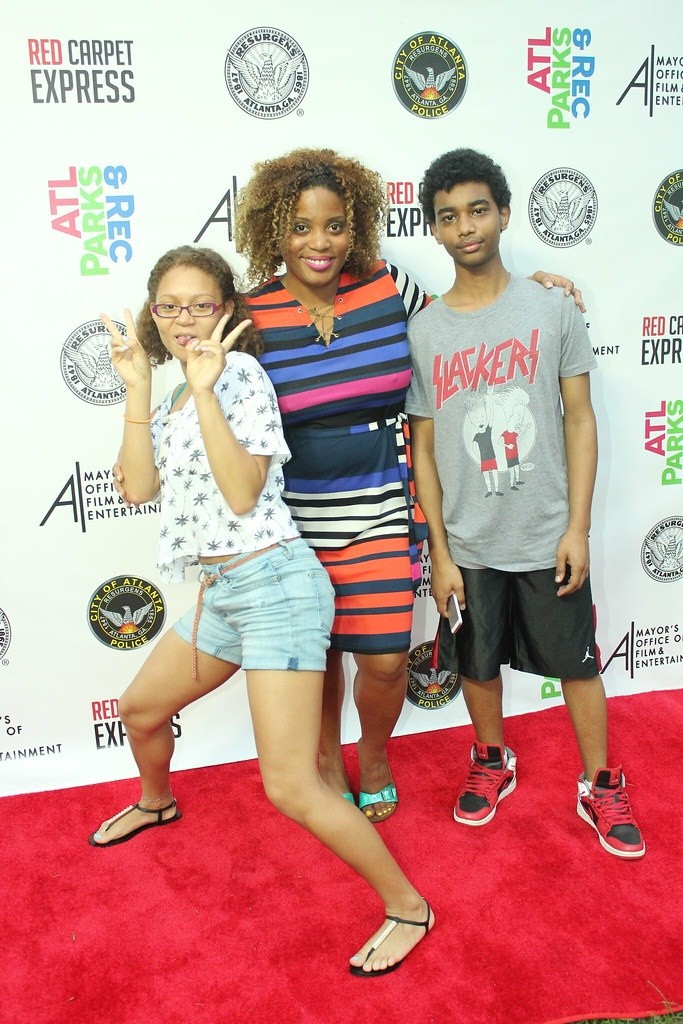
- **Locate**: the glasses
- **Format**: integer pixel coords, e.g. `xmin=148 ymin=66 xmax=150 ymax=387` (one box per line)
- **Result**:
xmin=152 ymin=303 xmax=225 ymax=317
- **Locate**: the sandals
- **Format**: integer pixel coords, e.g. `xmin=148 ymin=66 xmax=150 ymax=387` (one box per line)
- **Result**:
xmin=88 ymin=797 xmax=181 ymax=847
xmin=349 ymin=895 xmax=435 ymax=976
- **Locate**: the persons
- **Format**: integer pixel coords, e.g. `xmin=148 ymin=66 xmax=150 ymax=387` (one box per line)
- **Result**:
xmin=233 ymin=147 xmax=645 ymax=857
xmin=88 ymin=245 xmax=435 ymax=977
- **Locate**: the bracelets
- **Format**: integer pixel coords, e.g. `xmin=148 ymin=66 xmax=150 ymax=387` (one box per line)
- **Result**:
xmin=123 ymin=413 xmax=154 ymax=424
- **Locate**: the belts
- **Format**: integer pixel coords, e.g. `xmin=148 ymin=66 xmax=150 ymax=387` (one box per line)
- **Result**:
xmin=192 ymin=536 xmax=301 ymax=680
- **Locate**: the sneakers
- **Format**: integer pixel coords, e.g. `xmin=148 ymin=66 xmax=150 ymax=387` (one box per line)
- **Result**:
xmin=454 ymin=738 xmax=517 ymax=826
xmin=577 ymin=764 xmax=645 ymax=859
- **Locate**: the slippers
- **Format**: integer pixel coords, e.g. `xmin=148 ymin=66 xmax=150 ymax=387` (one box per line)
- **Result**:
xmin=358 ymin=736 xmax=398 ymax=822
xmin=340 ymin=791 xmax=355 ymax=805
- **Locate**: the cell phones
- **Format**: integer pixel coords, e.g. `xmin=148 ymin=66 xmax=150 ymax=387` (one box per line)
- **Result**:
xmin=447 ymin=594 xmax=462 ymax=634
